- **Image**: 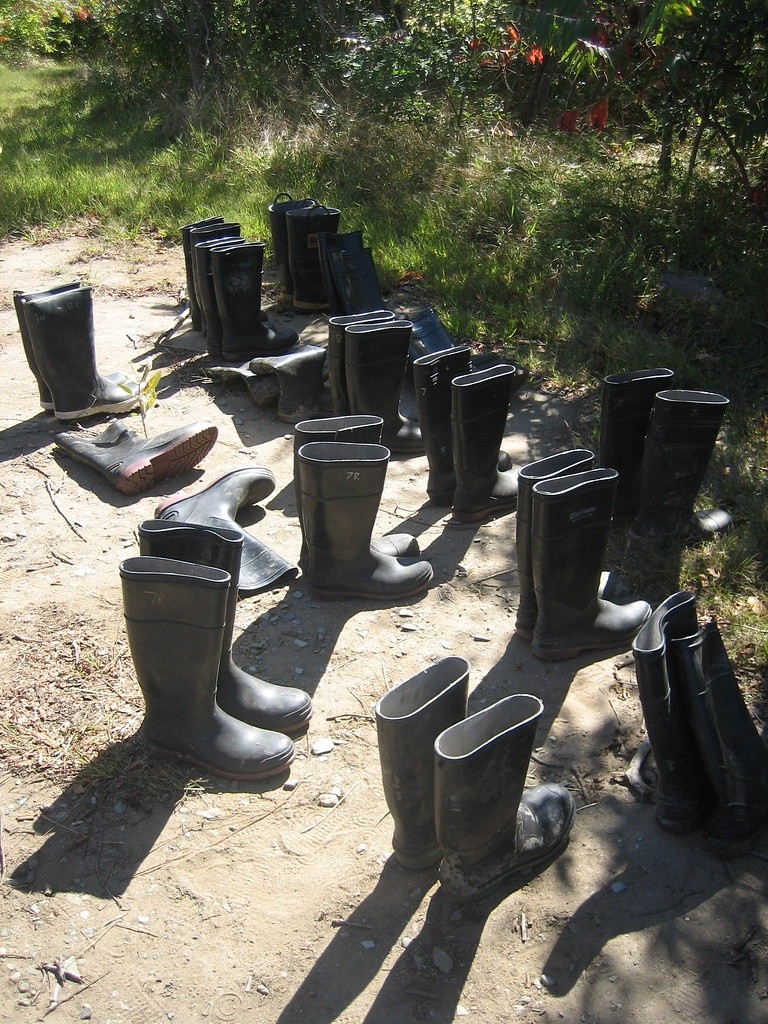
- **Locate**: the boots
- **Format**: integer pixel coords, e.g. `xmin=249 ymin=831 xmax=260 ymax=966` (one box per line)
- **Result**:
xmin=154 ymin=465 xmax=300 ymax=597
xmin=178 ymin=191 xmax=463 ymax=454
xmin=627 ymin=389 xmax=734 ymax=552
xmin=21 ymin=287 xmax=144 ymax=420
xmin=514 ymin=449 xmax=594 ymax=641
xmin=528 ymin=468 xmax=652 ymax=660
xmin=120 ymin=555 xmax=296 ymax=780
xmin=375 ymin=657 xmax=471 ymax=871
xmin=669 ymin=620 xmax=768 ymax=856
xmin=294 ymin=415 xmax=422 ymax=581
xmin=413 ymin=345 xmax=513 ymax=506
xmin=54 ymin=420 xmax=219 ymax=497
xmin=139 ymin=519 xmax=313 ymax=734
xmin=12 ymin=282 xmax=84 ymax=409
xmin=448 ymin=364 xmax=522 ymax=521
xmin=600 ymin=368 xmax=674 ymax=524
xmin=632 ymin=593 xmax=727 ymax=833
xmin=296 ymin=441 xmax=433 ymax=602
xmin=433 ymin=693 xmax=576 ymax=904
